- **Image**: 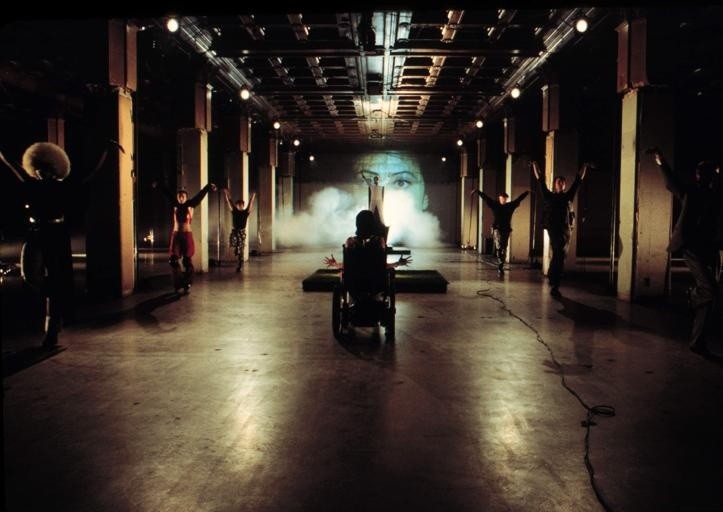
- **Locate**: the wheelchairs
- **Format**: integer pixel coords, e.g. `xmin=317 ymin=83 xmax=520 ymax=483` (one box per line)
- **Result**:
xmin=329 ymin=274 xmax=394 ymax=344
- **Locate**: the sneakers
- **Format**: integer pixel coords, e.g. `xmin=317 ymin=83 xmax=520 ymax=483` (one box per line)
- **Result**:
xmin=35 ymin=335 xmax=58 ymax=352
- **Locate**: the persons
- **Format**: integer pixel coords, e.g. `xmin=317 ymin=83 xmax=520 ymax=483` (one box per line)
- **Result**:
xmin=0 ymin=136 xmax=127 ymax=351
xmin=148 ymin=180 xmax=221 ymax=295
xmin=346 ymin=146 xmax=431 ymax=246
xmin=359 ymin=169 xmax=386 ymax=226
xmin=224 ymin=187 xmax=257 ymax=275
xmin=642 ymin=144 xmax=723 ymax=354
xmin=471 ymin=187 xmax=538 ymax=279
xmin=322 ymin=209 xmax=417 ymax=337
xmin=527 ymin=158 xmax=598 ymax=300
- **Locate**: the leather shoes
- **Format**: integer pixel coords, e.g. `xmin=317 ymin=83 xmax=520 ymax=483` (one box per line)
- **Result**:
xmin=683 ymin=286 xmax=697 ymax=321
xmin=688 ymin=344 xmax=721 ymax=361
